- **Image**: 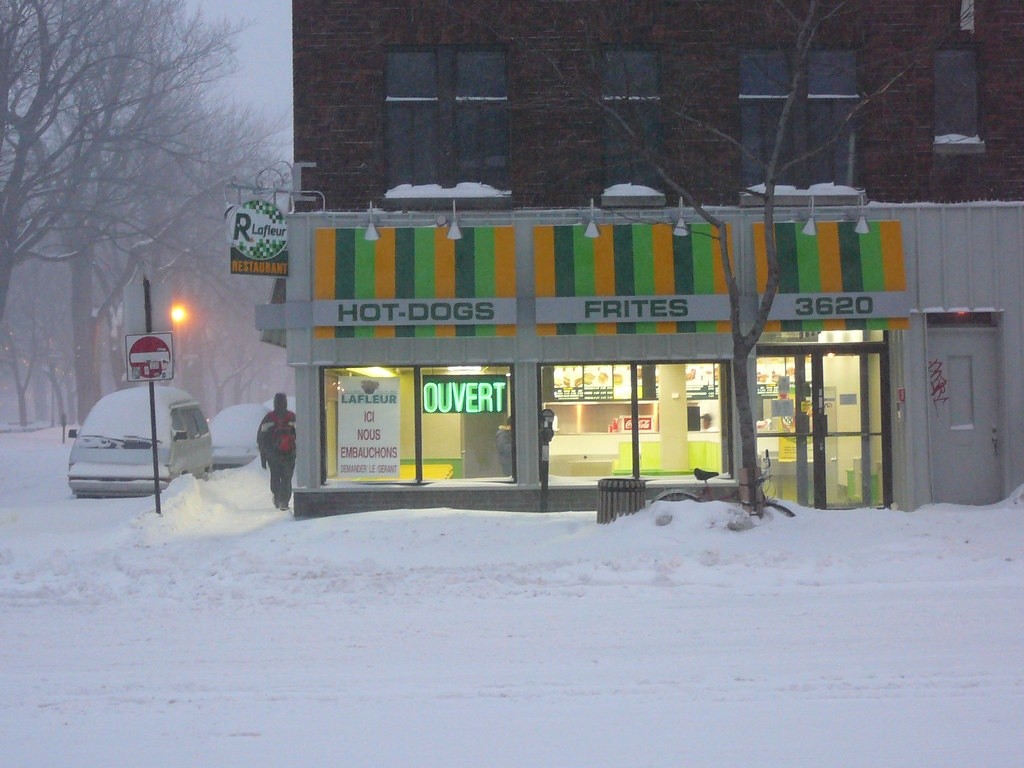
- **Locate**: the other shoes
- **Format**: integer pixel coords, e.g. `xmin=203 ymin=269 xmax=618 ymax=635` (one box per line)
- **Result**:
xmin=275 ymin=503 xmax=291 ymax=511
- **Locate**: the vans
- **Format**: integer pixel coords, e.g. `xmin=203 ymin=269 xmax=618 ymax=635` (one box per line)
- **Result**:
xmin=67 ymin=386 xmax=214 ymax=498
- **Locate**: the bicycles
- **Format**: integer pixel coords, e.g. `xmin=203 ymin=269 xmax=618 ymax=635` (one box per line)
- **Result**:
xmin=650 ymin=449 xmax=796 ymax=518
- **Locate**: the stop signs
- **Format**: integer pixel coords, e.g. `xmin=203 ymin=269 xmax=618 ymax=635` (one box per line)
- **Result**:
xmin=124 ymin=332 xmax=175 ymax=383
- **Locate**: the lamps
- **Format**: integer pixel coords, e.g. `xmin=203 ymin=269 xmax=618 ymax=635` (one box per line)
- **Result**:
xmin=673 ymin=197 xmax=689 ymax=236
xmin=584 ymin=198 xmax=601 ymax=238
xmin=448 ymin=199 xmax=462 ymax=240
xmin=800 ymin=196 xmax=818 ymax=235
xmin=364 ymin=199 xmax=381 ymax=241
xmin=856 ymin=195 xmax=870 ymax=235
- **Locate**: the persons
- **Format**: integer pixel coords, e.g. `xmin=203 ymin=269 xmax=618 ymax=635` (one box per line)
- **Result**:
xmin=257 ymin=392 xmax=297 ymax=511
xmin=700 ymin=414 xmax=719 ymax=432
xmin=495 ymin=415 xmax=513 ymax=477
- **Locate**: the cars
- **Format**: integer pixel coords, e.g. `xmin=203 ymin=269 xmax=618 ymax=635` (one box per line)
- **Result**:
xmin=208 ymin=397 xmax=298 ymax=470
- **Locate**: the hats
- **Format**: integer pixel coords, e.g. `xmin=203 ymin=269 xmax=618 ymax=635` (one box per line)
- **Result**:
xmin=273 ymin=393 xmax=285 ymax=402
